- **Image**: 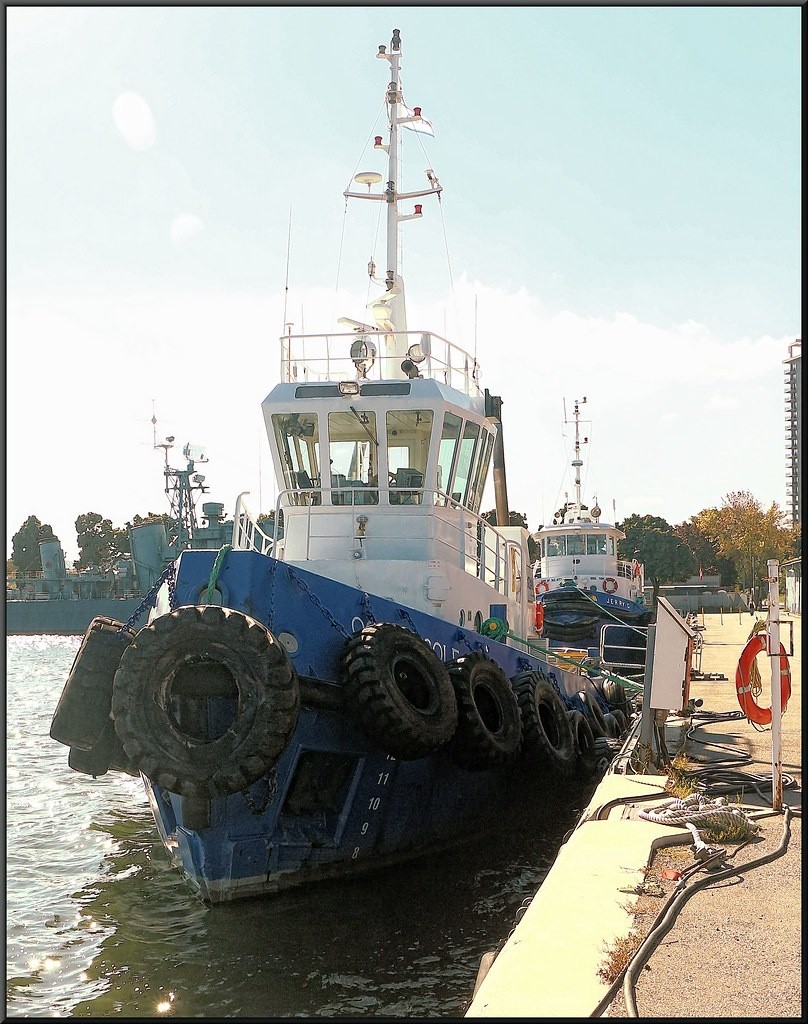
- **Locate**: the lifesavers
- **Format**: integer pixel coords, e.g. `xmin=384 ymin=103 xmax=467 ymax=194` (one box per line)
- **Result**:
xmin=603 ymin=577 xmax=618 ymax=594
xmin=534 ymin=582 xmax=549 ymax=594
xmin=566 ymin=709 xmax=599 ymax=777
xmin=638 ymin=611 xmax=653 ymax=632
xmin=441 ymin=652 xmax=525 ymax=772
xmin=68 ymin=714 xmax=117 ymax=777
xmin=736 ymin=632 xmax=792 ymax=725
xmin=342 ymin=621 xmax=459 ymax=760
xmin=590 ymin=736 xmax=623 ymax=773
xmin=535 ymin=600 xmax=544 ymax=636
xmin=111 ymin=601 xmax=302 ymax=798
xmin=509 ymin=668 xmax=577 ymax=774
xmin=574 ymin=690 xmax=610 ymax=737
xmin=108 ymin=735 xmax=142 ymax=778
xmin=50 ymin=614 xmax=136 ymax=753
xmin=603 ymin=677 xmax=634 ymax=736
xmin=634 ymin=560 xmax=639 ymax=574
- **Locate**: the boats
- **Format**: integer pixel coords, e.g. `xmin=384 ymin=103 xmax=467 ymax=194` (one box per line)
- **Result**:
xmin=7 ymin=28 xmax=656 ymax=906
